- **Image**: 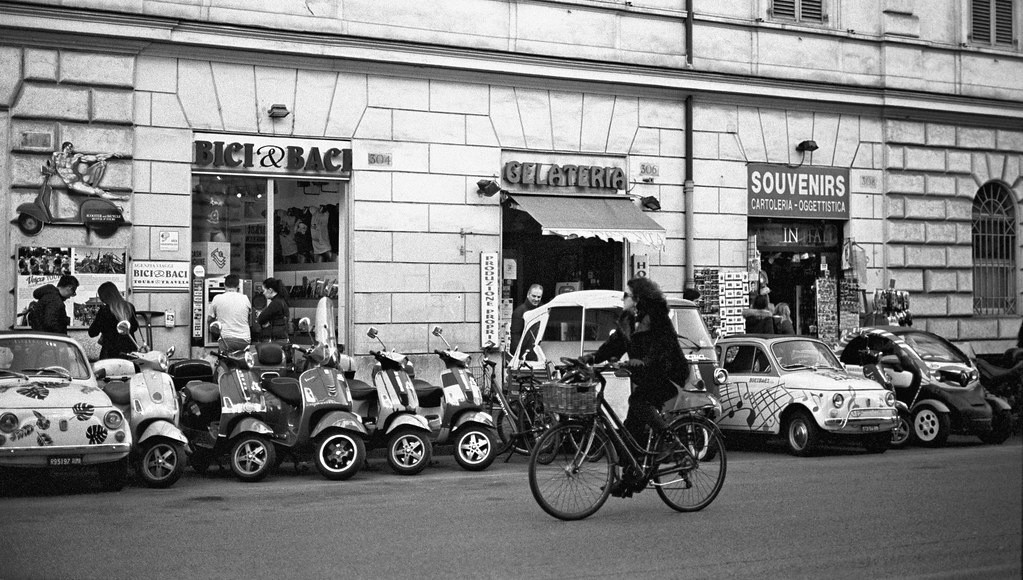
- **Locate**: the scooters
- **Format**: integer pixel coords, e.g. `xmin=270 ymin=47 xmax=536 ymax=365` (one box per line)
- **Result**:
xmin=336 ymin=327 xmax=434 ymax=475
xmin=253 ymin=317 xmax=372 ymax=481
xmin=166 ymin=320 xmax=276 ymax=483
xmin=970 ymin=344 xmax=1023 ymax=432
xmin=93 ymin=319 xmax=194 ymax=488
xmin=406 ymin=326 xmax=501 ymax=471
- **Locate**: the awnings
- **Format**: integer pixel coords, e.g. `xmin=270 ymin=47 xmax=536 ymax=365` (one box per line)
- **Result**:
xmin=511 ymin=194 xmax=666 ymax=246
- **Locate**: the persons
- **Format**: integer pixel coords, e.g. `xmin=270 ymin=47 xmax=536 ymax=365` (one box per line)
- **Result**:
xmin=257 ymin=278 xmax=290 ymax=340
xmin=87 ymin=282 xmax=138 ymax=360
xmin=579 ymin=277 xmax=689 ymax=494
xmin=683 ymin=287 xmax=700 ymax=304
xmin=750 ymin=281 xmax=757 ymax=294
xmin=208 ymin=274 xmax=252 ymax=353
xmin=33 ymin=275 xmax=79 ymax=335
xmin=772 ymin=302 xmax=794 ymax=334
xmin=18 ymin=254 xmax=69 ymax=273
xmin=743 ymin=294 xmax=774 ymax=334
xmin=510 ymin=284 xmax=544 ymax=361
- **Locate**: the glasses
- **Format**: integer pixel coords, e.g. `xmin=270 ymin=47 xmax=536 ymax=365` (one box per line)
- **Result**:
xmin=624 ymin=292 xmax=634 ymax=299
xmin=69 ymin=286 xmax=77 ymax=297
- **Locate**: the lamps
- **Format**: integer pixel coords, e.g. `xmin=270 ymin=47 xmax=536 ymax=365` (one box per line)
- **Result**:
xmin=271 ymin=102 xmax=291 ymax=119
xmin=795 ymin=140 xmax=821 ymax=153
xmin=476 ymin=179 xmax=502 ymax=197
xmin=639 ymin=197 xmax=662 ymax=210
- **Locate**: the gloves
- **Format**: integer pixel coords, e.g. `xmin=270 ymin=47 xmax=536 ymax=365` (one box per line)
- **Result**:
xmin=576 ymin=354 xmax=590 ymax=364
xmin=624 ymin=359 xmax=643 ymax=373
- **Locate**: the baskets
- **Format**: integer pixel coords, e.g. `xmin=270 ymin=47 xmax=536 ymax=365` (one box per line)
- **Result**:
xmin=468 ymin=366 xmax=489 ymax=391
xmin=543 ymin=381 xmax=597 ymax=416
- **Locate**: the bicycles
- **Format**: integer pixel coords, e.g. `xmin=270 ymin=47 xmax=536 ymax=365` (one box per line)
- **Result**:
xmin=468 ymin=338 xmax=612 ymax=464
xmin=527 ymin=356 xmax=729 ymax=522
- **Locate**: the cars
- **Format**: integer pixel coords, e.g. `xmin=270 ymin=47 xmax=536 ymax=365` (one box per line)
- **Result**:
xmin=0 ymin=329 xmax=133 ymax=494
xmin=712 ymin=331 xmax=900 ymax=457
xmin=832 ymin=326 xmax=1015 ymax=449
xmin=506 ymin=289 xmax=721 ymax=449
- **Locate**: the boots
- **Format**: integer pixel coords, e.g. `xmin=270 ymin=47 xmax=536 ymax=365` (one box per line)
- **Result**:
xmin=601 ymin=460 xmax=641 ymax=498
xmin=650 ymin=415 xmax=681 ymax=461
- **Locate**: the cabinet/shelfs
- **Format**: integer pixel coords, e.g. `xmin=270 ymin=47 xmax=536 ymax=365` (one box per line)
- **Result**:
xmin=273 ymin=262 xmax=344 ymax=345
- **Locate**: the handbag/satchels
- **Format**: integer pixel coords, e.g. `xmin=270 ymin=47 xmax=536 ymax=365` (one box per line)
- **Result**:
xmin=28 ymin=304 xmax=46 ymax=332
xmin=261 ymin=302 xmax=288 ymax=339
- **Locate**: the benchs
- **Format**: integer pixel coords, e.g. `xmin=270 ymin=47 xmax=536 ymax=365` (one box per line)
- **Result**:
xmin=541 ymin=339 xmax=630 ymax=378
